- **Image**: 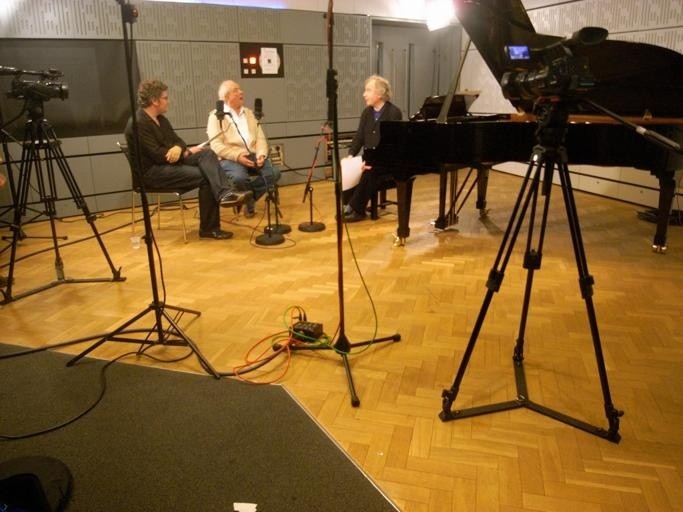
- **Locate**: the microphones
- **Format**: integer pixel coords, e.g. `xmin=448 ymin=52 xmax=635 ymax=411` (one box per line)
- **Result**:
xmin=253 ymin=98 xmax=264 ymax=118
xmin=217 ymin=100 xmax=225 ymax=121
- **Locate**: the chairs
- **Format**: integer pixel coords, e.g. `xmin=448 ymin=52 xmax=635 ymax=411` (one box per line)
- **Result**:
xmin=116 ymin=137 xmax=199 ymax=245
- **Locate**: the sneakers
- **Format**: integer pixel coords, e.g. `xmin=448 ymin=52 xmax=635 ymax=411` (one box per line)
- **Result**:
xmin=198 ymin=229 xmax=234 ymax=241
xmin=218 ymin=189 xmax=257 ymax=219
xmin=334 ymin=203 xmax=370 ymax=224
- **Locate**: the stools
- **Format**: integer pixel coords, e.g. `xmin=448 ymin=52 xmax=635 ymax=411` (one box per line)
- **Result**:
xmin=362 ymin=174 xmax=398 ymax=221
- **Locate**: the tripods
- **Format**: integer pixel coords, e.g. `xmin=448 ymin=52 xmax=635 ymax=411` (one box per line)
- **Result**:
xmin=271 ymin=68 xmax=401 ymax=406
xmin=68 ymin=25 xmax=222 ymax=382
xmin=0 ymin=122 xmax=125 ymax=306
xmin=440 ymin=144 xmax=625 ymax=443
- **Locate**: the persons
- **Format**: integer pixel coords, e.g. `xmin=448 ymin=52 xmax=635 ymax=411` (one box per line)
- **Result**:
xmin=123 ymin=80 xmax=255 ymax=240
xmin=334 ymin=75 xmax=403 ymax=224
xmin=203 ymin=81 xmax=280 ymax=218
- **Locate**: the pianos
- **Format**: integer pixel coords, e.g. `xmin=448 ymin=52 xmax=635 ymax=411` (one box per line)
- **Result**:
xmin=376 ymin=1 xmax=683 ymax=254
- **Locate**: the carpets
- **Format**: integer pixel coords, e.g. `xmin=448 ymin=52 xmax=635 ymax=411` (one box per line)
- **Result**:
xmin=0 ymin=330 xmax=401 ymax=511
